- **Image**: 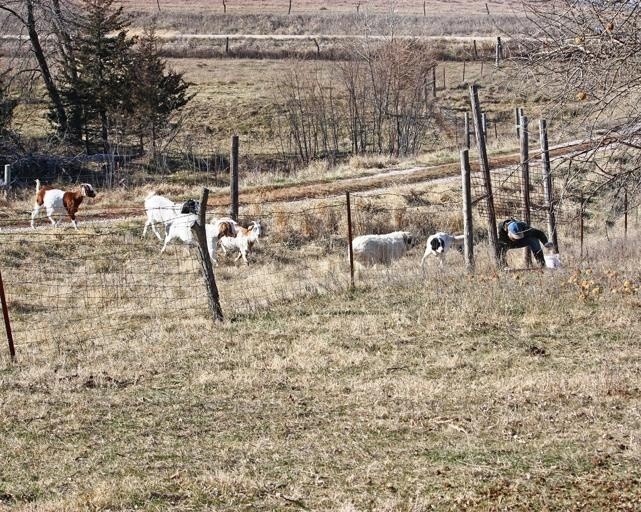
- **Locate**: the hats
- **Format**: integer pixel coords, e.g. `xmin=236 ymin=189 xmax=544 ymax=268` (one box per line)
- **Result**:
xmin=507 ymin=221 xmax=524 ymax=240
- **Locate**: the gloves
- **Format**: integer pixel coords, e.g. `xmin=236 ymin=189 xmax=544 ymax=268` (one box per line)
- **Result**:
xmin=544 ymin=242 xmax=554 ymax=249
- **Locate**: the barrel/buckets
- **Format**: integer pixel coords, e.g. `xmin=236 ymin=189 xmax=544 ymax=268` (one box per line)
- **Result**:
xmin=544 ymin=253 xmax=561 ymax=269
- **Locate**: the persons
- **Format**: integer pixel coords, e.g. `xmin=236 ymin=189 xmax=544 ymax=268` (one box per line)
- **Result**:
xmin=484 ymin=219 xmax=553 ymax=272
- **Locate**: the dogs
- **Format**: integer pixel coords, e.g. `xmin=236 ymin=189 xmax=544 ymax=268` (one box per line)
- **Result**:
xmin=421 ymin=232 xmax=454 ymax=265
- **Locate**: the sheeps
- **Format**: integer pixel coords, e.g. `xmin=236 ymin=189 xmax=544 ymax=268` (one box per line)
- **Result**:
xmin=157 ymin=213 xmax=239 ymax=266
xmin=30 ymin=178 xmax=96 ymax=231
xmin=348 ymin=231 xmax=414 ymax=271
xmin=141 ymin=190 xmax=201 ymax=241
xmin=209 ymin=217 xmax=266 ymax=266
